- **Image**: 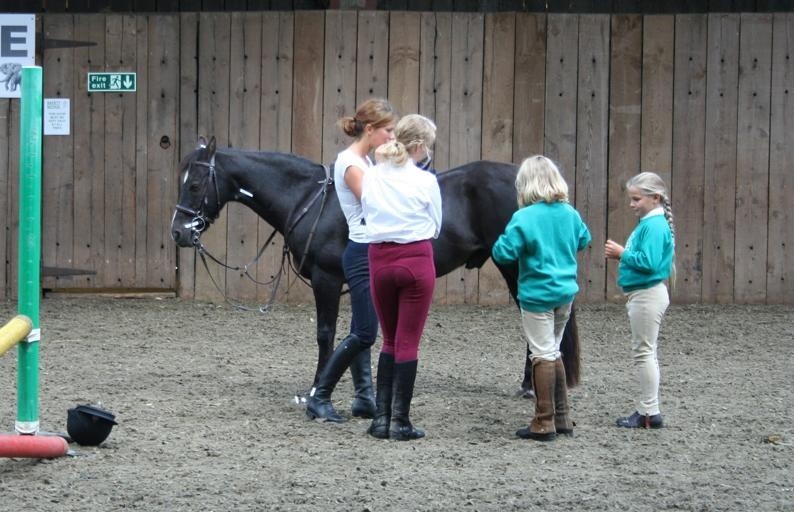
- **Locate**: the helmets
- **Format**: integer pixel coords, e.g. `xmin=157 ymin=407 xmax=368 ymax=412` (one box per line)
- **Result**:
xmin=66 ymin=403 xmax=118 ymax=446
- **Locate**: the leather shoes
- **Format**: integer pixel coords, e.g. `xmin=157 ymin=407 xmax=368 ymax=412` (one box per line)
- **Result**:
xmin=615 ymin=413 xmax=663 ymax=430
xmin=558 ymin=426 xmax=574 ymax=437
xmin=516 ymin=429 xmax=556 ymax=444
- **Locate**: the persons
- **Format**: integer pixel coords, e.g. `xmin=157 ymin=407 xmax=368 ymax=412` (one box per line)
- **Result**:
xmin=361 ymin=113 xmax=442 ymax=442
xmin=304 ymin=97 xmax=395 ymax=423
xmin=491 ymin=155 xmax=592 ymax=442
xmin=603 ymin=170 xmax=676 ymax=429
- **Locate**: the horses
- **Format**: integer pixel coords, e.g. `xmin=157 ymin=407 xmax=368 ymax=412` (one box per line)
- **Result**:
xmin=169 ymin=134 xmax=583 ymax=399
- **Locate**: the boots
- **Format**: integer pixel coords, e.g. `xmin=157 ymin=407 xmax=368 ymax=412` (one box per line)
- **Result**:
xmin=388 ymin=361 xmax=424 ymax=442
xmin=366 ymin=352 xmax=393 ymax=439
xmin=350 ymin=351 xmax=376 ymax=419
xmin=304 ymin=334 xmax=359 ymax=423
